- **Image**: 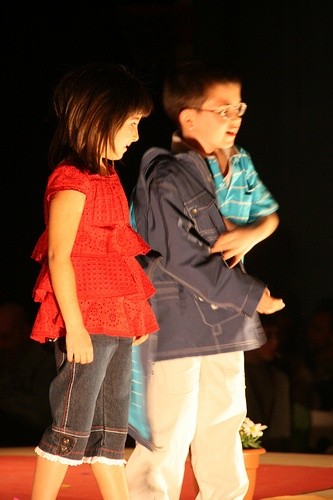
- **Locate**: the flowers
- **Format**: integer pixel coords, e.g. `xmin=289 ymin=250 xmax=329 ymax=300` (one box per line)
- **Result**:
xmin=239 ymin=417 xmax=268 ymax=449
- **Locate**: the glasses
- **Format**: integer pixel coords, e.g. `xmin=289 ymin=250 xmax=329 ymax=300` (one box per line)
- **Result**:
xmin=191 ymin=102 xmax=247 ymax=121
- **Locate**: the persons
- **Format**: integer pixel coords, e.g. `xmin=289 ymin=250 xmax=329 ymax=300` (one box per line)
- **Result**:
xmin=244 ymin=290 xmax=333 ymax=455
xmin=124 ymin=60 xmax=285 ymax=500
xmin=29 ymin=60 xmax=162 ymax=500
xmin=0 ymin=301 xmax=56 ymax=447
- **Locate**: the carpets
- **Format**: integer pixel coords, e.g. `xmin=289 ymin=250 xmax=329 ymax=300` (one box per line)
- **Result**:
xmin=0 ymin=455 xmax=333 ymax=500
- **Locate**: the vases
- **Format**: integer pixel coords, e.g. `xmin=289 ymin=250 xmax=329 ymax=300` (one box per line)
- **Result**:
xmin=243 ymin=448 xmax=266 ymax=500
